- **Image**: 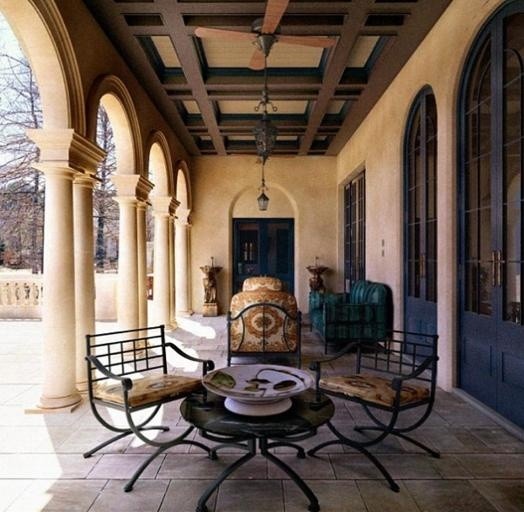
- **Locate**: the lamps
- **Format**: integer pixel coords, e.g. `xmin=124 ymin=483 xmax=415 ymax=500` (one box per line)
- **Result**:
xmin=250 ymin=33 xmax=277 ymax=211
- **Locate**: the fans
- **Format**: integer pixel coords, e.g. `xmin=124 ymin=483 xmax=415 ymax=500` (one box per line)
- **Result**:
xmin=193 ymin=1 xmax=338 ymax=72
